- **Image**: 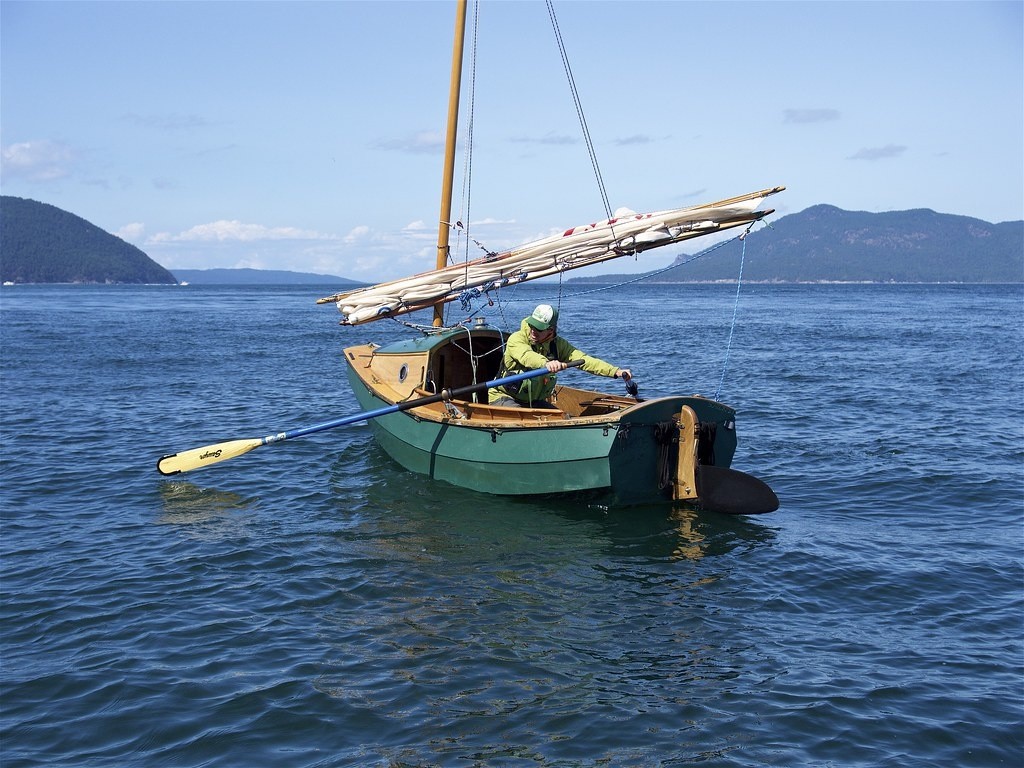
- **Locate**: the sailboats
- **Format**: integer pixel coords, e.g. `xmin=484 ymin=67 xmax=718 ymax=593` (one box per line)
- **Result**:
xmin=316 ymin=1 xmax=787 ymax=517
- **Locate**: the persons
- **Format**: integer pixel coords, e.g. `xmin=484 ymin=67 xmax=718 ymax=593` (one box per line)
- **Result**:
xmin=487 ymin=304 xmax=632 ymax=409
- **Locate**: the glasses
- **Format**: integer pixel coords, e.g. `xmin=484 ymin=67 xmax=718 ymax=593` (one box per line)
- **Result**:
xmin=528 ymin=323 xmax=543 ymax=332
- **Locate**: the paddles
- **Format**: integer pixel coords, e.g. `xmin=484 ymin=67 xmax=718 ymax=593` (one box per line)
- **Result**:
xmin=157 ymin=356 xmax=588 ymax=478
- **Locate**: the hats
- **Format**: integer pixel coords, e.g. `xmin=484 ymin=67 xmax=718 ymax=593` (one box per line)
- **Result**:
xmin=526 ymin=304 xmax=558 ymax=330
